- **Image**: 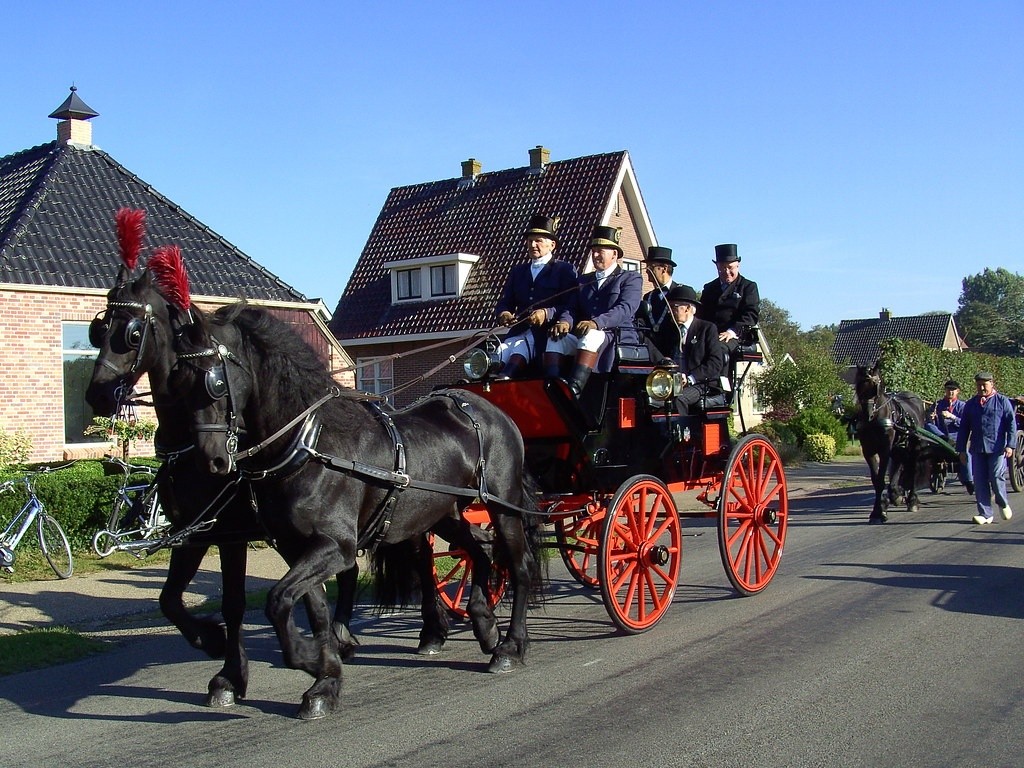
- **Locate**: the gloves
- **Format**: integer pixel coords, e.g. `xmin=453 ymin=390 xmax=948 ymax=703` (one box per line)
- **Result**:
xmin=574 ymin=320 xmax=597 ymax=339
xmin=498 ymin=311 xmax=518 ymax=327
xmin=527 ymin=309 xmax=546 ymax=328
xmin=550 ymin=320 xmax=570 ymax=337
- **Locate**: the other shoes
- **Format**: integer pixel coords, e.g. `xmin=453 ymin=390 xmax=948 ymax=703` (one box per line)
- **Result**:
xmin=940 ymin=436 xmax=955 ymax=447
xmin=966 ymin=482 xmax=975 ymax=495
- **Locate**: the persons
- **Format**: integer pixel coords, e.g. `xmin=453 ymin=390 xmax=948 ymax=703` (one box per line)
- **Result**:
xmin=643 ymin=246 xmax=684 ymax=362
xmin=927 ymin=380 xmax=977 ymax=497
xmin=694 ymin=244 xmax=760 ymax=391
xmin=486 ymin=210 xmax=580 ymax=382
xmin=655 ymin=284 xmax=725 ymax=472
xmin=543 ymin=226 xmax=643 ymax=420
xmin=954 ymin=372 xmax=1016 ymax=524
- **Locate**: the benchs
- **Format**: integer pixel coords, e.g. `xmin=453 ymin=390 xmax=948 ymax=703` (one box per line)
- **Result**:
xmin=732 ymin=327 xmax=764 ymax=362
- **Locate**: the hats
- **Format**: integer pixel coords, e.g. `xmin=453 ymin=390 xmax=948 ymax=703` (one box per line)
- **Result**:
xmin=945 ymin=380 xmax=959 ymax=389
xmin=975 ymin=373 xmax=992 ymax=380
xmin=712 ymin=244 xmax=741 ymax=264
xmin=586 ymin=226 xmax=624 ymax=259
xmin=666 ymin=285 xmax=701 ymax=306
xmin=641 ymin=247 xmax=677 ymax=267
xmin=523 ymin=215 xmax=561 ymax=254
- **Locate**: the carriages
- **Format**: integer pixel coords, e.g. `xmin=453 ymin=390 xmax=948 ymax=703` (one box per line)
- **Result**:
xmin=82 ymin=208 xmax=791 ymax=720
xmin=834 ymin=360 xmax=1024 ymax=526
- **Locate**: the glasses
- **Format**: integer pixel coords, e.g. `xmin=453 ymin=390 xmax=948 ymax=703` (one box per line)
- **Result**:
xmin=647 ymin=264 xmax=663 ymax=272
xmin=668 ymin=303 xmax=690 ymax=311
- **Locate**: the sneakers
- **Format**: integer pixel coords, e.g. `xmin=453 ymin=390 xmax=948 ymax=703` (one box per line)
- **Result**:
xmin=973 ymin=516 xmax=993 ymax=524
xmin=999 ymin=504 xmax=1012 ymax=520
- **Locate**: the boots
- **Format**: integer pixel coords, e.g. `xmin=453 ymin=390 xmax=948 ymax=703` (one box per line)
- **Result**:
xmin=494 ymin=353 xmax=526 ymax=382
xmin=540 ymin=350 xmax=599 ymax=401
xmin=488 ymin=361 xmax=505 ymax=378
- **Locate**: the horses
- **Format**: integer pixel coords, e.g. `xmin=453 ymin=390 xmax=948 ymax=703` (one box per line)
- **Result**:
xmin=85 ymin=264 xmax=550 ymax=721
xmin=855 ymin=360 xmax=928 ymax=525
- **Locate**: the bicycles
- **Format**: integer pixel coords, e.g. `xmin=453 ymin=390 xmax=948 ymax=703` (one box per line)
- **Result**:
xmin=99 ymin=454 xmax=174 ymax=560
xmin=0 ymin=466 xmax=74 ymax=580
